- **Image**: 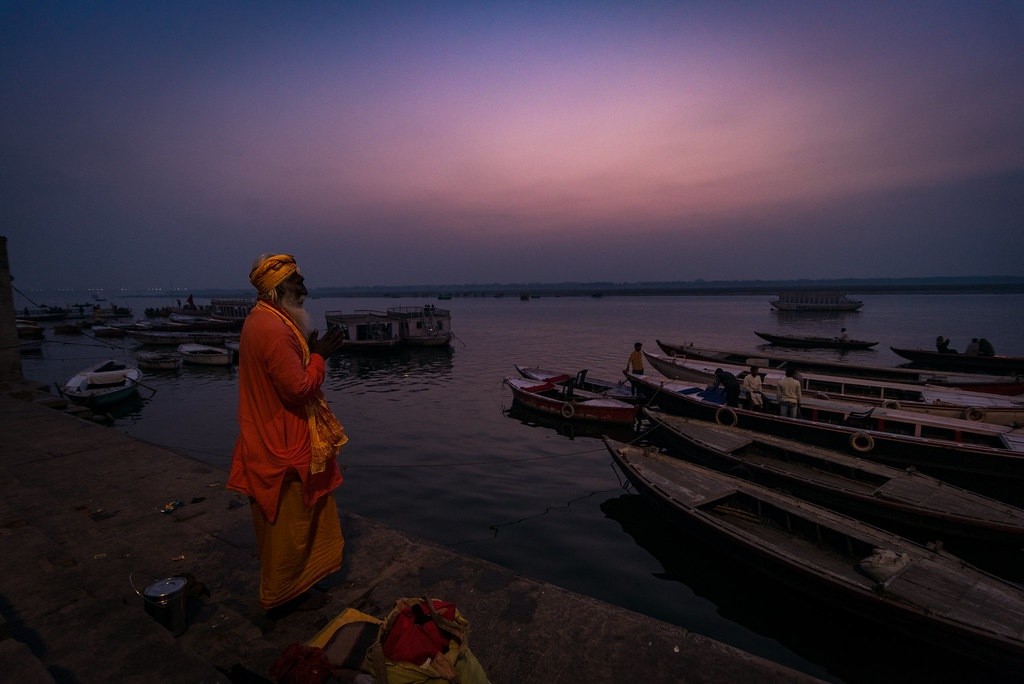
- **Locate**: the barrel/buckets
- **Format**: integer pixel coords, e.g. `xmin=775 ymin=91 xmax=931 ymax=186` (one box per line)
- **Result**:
xmin=130 ymin=570 xmax=188 ymax=638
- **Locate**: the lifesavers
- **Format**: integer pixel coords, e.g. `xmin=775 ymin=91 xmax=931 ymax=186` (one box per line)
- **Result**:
xmin=849 ymin=432 xmax=875 ymax=453
xmin=562 ymin=404 xmax=574 ymax=418
xmin=813 ymin=394 xmax=829 ymax=400
xmin=882 ymin=399 xmax=901 ymax=410
xmin=729 ymin=465 xmax=754 ymax=481
xmin=964 ymin=407 xmax=987 ymax=422
xmin=716 ymin=407 xmax=738 ymax=426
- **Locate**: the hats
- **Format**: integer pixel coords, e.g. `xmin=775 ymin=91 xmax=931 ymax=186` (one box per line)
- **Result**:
xmin=251 ymin=255 xmax=297 ymax=292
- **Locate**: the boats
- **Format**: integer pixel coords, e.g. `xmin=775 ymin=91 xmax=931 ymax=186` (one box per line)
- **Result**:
xmin=768 ymin=289 xmax=866 ymax=311
xmin=887 ymin=345 xmax=1024 ymax=377
xmin=222 ymin=335 xmax=240 ymax=364
xmin=600 ymin=339 xmax=1024 ymax=678
xmin=60 ymin=358 xmax=144 ymax=413
xmin=513 ymin=363 xmax=646 ymax=406
xmin=501 ymin=375 xmax=640 ymax=427
xmin=14 ymin=299 xmax=256 ymax=346
xmin=753 ymin=330 xmax=881 ymax=351
xmin=133 ymin=347 xmax=182 ymax=371
xmin=175 ymin=342 xmax=235 ymax=366
xmin=386 ymin=305 xmax=455 ymax=350
xmin=324 ymin=309 xmax=402 ymax=355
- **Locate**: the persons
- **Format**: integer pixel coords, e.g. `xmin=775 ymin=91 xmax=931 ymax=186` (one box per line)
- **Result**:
xmin=743 ymin=365 xmax=763 ymax=412
xmin=966 ymin=337 xmax=996 ymax=356
xmin=225 ymin=255 xmax=349 ymax=610
xmin=936 ymin=336 xmax=959 ymax=354
xmin=177 ymin=299 xmax=180 ymax=309
xmin=626 ymin=342 xmax=644 ymax=397
xmin=715 ymin=368 xmax=741 ymax=407
xmin=835 ymin=328 xmax=849 ymax=342
xmin=776 ymin=367 xmax=802 ymax=418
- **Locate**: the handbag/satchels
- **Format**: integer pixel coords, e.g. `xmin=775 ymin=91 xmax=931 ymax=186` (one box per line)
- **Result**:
xmin=358 ymin=597 xmax=491 ymax=684
